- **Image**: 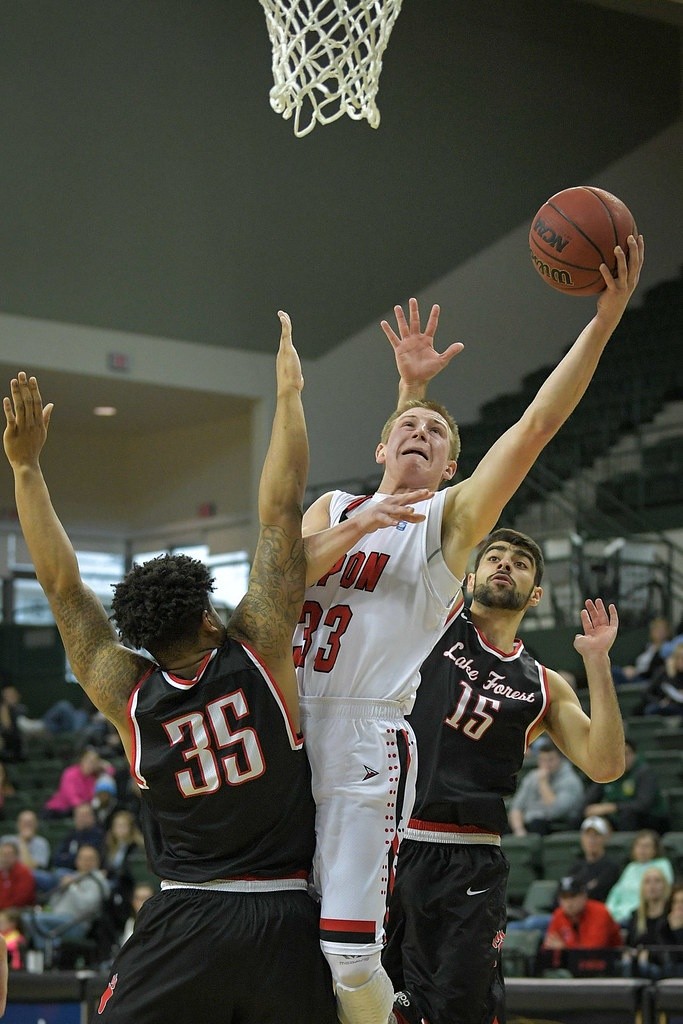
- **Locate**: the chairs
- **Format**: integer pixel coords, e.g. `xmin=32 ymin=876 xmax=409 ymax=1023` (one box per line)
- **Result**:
xmin=1 ymin=664 xmax=683 ymax=976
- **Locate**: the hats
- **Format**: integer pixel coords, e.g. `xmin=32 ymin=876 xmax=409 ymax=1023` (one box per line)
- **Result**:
xmin=559 ymin=875 xmax=586 ymax=894
xmin=583 ymin=815 xmax=610 ymax=835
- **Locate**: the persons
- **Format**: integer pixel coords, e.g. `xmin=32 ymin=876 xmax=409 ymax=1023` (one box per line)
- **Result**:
xmin=0 ymin=686 xmax=154 ymax=974
xmin=507 ymin=745 xmax=586 ymax=837
xmin=41 ymin=747 xmax=117 ymax=819
xmin=292 ymin=231 xmax=645 ymax=1024
xmin=379 ymin=297 xmax=627 ymax=1024
xmin=570 ymin=739 xmax=656 ymax=831
xmin=618 ymin=869 xmax=683 ymax=981
xmin=604 ymin=828 xmax=674 ymax=927
xmin=2 ymin=312 xmax=345 ymax=1024
xmin=551 ymin=816 xmax=622 ymax=911
xmin=609 ymin=615 xmax=683 ymax=718
xmin=0 ymin=809 xmax=50 ymax=870
xmin=539 ymin=874 xmax=624 ymax=979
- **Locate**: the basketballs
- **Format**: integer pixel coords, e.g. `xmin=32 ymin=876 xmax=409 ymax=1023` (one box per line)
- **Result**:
xmin=527 ymin=185 xmax=639 ymax=298
